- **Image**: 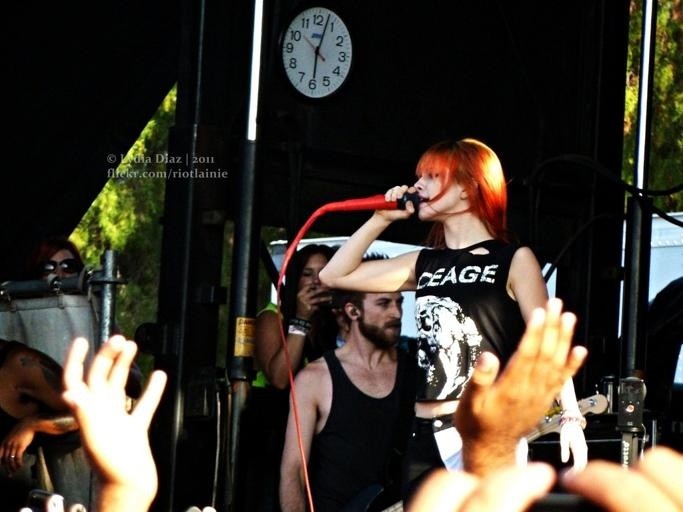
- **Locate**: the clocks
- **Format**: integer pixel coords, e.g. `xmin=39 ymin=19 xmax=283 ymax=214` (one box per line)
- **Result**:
xmin=271 ymin=6 xmax=358 ymax=107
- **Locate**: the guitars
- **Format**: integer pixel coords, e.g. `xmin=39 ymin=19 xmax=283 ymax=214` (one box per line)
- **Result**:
xmin=365 ymin=394 xmax=608 ymax=511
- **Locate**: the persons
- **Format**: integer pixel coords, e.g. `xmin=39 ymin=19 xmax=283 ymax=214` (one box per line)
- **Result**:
xmin=278 ymin=253 xmax=417 ymax=512
xmin=319 ymin=138 xmax=588 ymax=479
xmin=1 ymin=338 xmax=81 ymax=512
xmin=231 ymin=244 xmax=345 ymax=512
xmin=32 ymin=237 xmax=84 ymax=280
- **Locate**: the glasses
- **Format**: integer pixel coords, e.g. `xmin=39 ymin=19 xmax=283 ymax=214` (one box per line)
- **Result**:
xmin=43 ymin=258 xmax=82 ymax=274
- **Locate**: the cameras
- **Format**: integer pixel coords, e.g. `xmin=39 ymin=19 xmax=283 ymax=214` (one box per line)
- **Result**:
xmin=308 ymin=288 xmax=337 ymax=308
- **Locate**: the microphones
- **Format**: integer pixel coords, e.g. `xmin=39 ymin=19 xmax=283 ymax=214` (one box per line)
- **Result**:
xmin=326 ymin=190 xmax=419 ymax=215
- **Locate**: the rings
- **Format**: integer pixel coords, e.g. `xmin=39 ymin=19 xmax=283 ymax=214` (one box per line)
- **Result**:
xmin=10 ymin=456 xmax=16 ymax=459
